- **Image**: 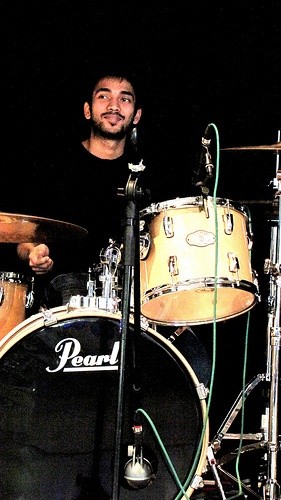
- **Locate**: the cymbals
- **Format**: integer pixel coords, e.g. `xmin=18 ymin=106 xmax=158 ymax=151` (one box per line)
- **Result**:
xmin=219 ymin=141 xmax=281 ymax=150
xmin=0 ymin=211 xmax=88 ymax=243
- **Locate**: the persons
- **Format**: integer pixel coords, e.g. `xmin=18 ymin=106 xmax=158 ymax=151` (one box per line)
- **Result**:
xmin=16 ymin=74 xmax=211 ymax=386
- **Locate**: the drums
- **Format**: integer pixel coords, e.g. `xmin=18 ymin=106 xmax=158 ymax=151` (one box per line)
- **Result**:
xmin=0 ymin=271 xmax=35 ymax=352
xmin=139 ymin=196 xmax=261 ymax=327
xmin=0 ymin=295 xmax=210 ymax=500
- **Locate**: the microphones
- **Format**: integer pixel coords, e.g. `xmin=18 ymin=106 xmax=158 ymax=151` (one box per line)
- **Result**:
xmin=124 ymin=410 xmax=154 ymax=489
xmin=192 ymin=124 xmax=213 ymax=185
